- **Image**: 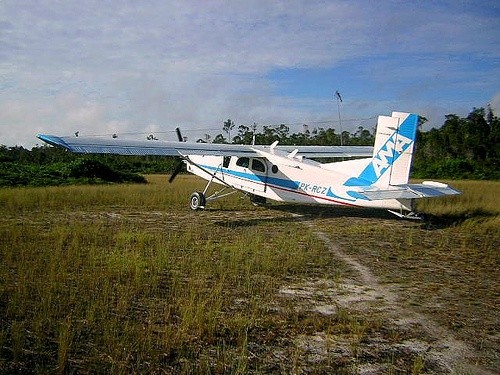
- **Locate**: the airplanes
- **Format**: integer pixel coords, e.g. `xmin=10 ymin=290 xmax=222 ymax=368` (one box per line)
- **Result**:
xmin=36 ymin=110 xmax=461 ymax=221
